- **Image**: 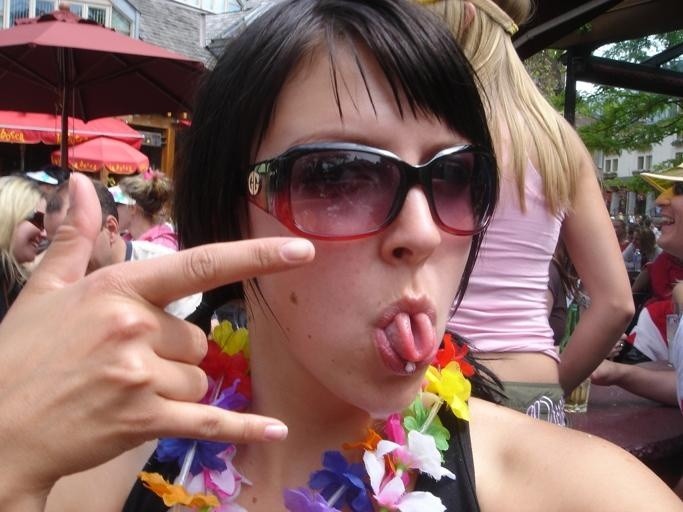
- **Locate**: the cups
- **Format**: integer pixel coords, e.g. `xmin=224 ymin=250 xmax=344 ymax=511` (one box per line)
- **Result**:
xmin=564 ymin=377 xmax=593 ymax=414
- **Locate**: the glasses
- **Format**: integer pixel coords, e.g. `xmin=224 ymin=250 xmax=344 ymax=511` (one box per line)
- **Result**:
xmin=671 ymin=183 xmax=683 ymax=195
xmin=23 ymin=211 xmax=45 ymax=232
xmin=246 ymin=142 xmax=499 ymax=240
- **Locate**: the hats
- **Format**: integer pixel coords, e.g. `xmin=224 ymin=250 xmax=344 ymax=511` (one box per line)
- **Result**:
xmin=105 ymin=184 xmax=138 ymax=206
xmin=639 ymin=158 xmax=683 ymax=193
xmin=27 ymin=169 xmax=60 ymax=187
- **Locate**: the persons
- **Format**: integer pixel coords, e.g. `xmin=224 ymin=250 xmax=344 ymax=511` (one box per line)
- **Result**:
xmin=548 ymin=169 xmax=683 ymax=405
xmin=0 ymin=172 xmax=204 ymax=324
xmin=0 ymin=0 xmax=683 ymax=512
xmin=418 ymin=0 xmax=635 ymax=428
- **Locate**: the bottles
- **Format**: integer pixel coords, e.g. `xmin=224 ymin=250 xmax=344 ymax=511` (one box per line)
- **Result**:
xmin=557 ymin=304 xmax=580 ymax=351
xmin=633 ymin=249 xmax=642 ymax=271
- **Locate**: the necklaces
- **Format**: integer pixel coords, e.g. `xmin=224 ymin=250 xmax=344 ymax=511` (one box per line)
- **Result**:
xmin=137 ymin=320 xmax=476 ymax=512
